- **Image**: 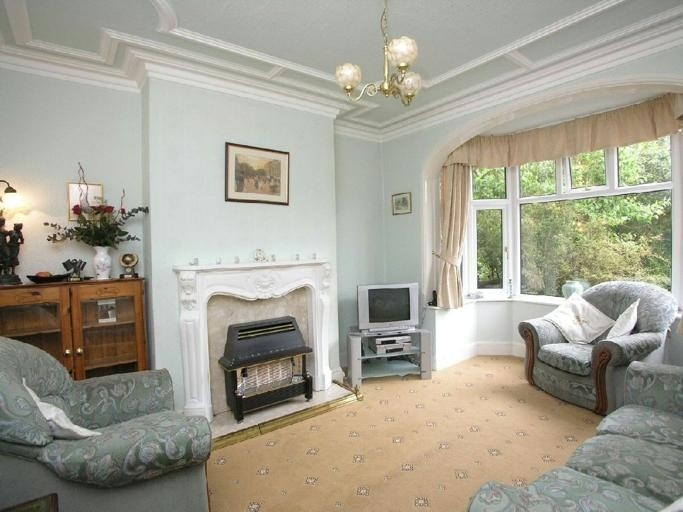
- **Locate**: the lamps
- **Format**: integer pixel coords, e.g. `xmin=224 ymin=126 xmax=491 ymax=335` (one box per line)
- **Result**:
xmin=335 ymin=0 xmax=421 ymax=107
xmin=0 ymin=179 xmax=16 ymax=193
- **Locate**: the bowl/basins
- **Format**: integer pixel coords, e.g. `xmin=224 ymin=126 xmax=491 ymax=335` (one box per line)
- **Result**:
xmin=26 ymin=273 xmax=73 ymax=283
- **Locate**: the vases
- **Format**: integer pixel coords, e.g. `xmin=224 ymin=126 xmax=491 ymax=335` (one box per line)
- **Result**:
xmin=91 ymin=246 xmax=112 ymax=280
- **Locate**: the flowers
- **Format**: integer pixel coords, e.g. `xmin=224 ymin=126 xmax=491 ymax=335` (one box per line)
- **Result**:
xmin=42 ymin=162 xmax=149 ymax=250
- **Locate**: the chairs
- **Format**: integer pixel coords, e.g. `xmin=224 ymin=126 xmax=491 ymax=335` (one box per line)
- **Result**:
xmin=517 ymin=280 xmax=679 ymax=417
xmin=0 ymin=335 xmax=211 ymax=512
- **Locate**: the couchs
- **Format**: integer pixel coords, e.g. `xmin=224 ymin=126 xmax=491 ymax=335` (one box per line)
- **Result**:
xmin=468 ymin=361 xmax=683 ymax=512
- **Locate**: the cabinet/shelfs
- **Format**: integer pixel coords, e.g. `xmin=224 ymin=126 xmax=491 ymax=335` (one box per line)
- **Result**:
xmin=0 ymin=277 xmax=149 ymax=381
xmin=347 ymin=329 xmax=432 ymax=390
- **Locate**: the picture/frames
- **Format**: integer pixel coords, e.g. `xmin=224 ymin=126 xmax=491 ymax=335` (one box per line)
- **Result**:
xmin=68 ymin=183 xmax=103 ymax=221
xmin=392 ymin=192 xmax=412 ymax=215
xmin=225 ymin=142 xmax=289 ymax=205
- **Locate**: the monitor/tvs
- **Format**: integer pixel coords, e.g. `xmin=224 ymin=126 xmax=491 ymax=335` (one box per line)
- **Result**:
xmin=358 ymin=282 xmax=418 ymax=330
